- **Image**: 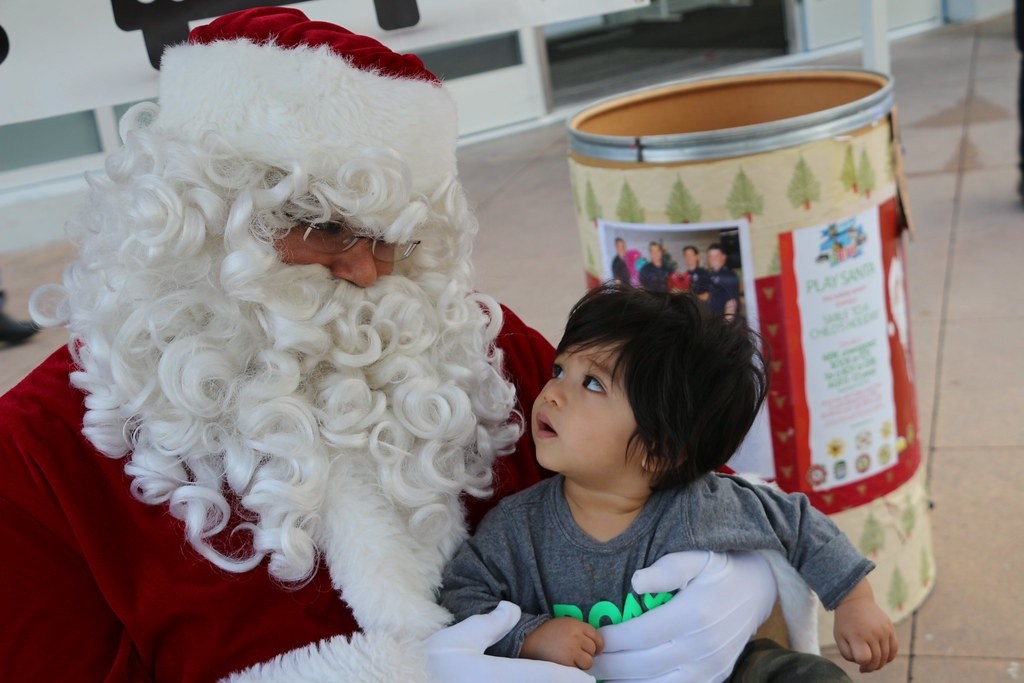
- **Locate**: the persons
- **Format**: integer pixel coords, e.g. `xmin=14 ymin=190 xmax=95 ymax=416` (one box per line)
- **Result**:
xmin=435 ymin=282 xmax=900 ymax=683
xmin=610 ymin=238 xmax=740 ymax=325
xmin=0 ymin=7 xmax=821 ymax=683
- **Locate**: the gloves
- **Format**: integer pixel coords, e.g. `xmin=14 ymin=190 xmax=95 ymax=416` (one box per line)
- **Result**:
xmin=580 ymin=549 xmax=779 ymax=683
xmin=421 ymin=600 xmax=596 ymax=683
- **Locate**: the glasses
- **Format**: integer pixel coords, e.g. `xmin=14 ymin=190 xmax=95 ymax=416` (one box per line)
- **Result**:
xmin=284 ymin=211 xmax=421 ymax=263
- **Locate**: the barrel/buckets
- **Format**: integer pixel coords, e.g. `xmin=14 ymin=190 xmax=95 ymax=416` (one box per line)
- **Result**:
xmin=566 ymin=64 xmax=936 ymax=648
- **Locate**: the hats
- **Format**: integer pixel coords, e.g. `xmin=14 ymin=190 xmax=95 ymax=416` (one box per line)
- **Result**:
xmin=157 ymin=5 xmax=460 ymax=239
